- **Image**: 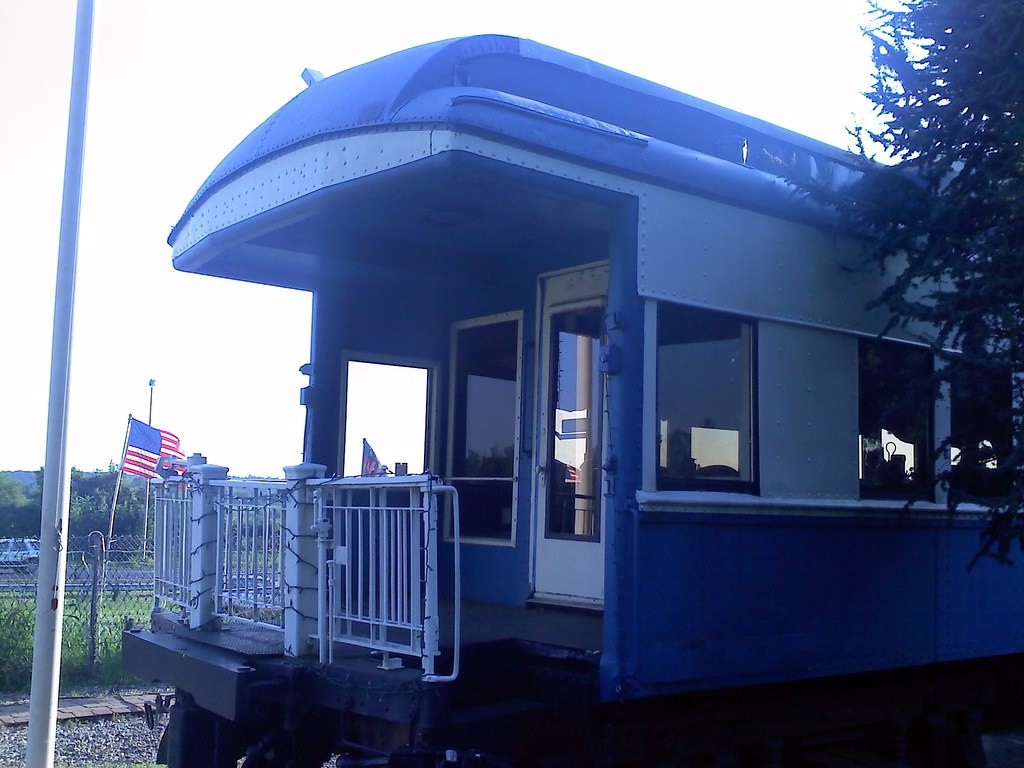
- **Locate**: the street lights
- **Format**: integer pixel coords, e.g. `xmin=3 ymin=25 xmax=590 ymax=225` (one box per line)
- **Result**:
xmin=141 ymin=379 xmax=155 ymax=559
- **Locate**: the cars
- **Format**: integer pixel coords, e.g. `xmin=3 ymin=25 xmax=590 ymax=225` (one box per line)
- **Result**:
xmin=0 ymin=539 xmax=41 ymax=574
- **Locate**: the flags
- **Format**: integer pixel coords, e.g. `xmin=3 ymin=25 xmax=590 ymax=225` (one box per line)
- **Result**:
xmin=361 ymin=441 xmax=386 ymax=477
xmin=566 ymin=464 xmax=582 ymax=481
xmin=118 ymin=417 xmax=187 ymax=488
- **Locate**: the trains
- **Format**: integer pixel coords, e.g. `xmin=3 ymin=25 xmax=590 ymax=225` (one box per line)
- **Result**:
xmin=121 ymin=34 xmax=1024 ymax=768
